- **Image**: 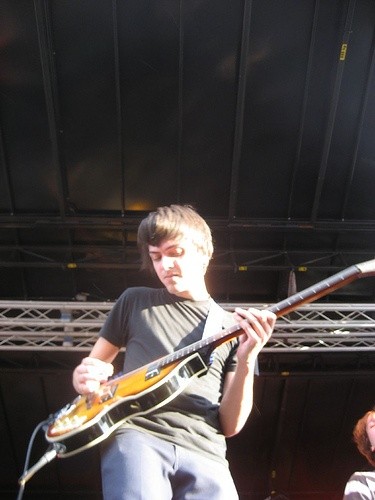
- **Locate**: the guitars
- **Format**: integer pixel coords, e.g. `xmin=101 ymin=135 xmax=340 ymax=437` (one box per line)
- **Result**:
xmin=44 ymin=259 xmax=375 ymax=458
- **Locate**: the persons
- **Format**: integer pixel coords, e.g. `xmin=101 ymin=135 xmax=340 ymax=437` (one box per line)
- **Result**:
xmin=340 ymin=409 xmax=375 ymax=500
xmin=72 ymin=204 xmax=278 ymax=500
